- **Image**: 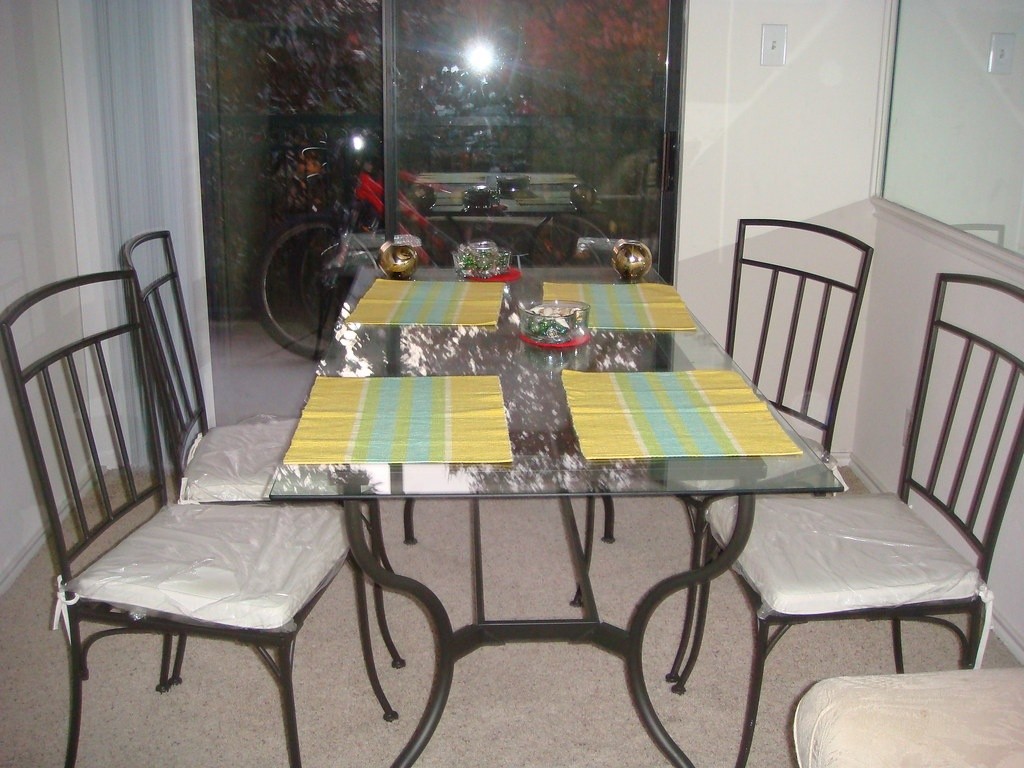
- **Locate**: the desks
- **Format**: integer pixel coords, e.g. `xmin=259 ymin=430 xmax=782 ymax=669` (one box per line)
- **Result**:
xmin=271 ymin=267 xmax=844 ymax=768
xmin=409 ymin=171 xmax=602 ymax=265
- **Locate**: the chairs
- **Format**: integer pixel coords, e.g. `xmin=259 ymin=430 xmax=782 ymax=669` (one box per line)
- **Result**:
xmin=0 ymin=269 xmax=401 ymax=768
xmin=569 ymin=219 xmax=873 ymax=683
xmin=121 ymin=229 xmax=406 ymax=685
xmin=673 ymin=273 xmax=1024 ymax=768
xmin=792 ymin=668 xmax=1024 ymax=768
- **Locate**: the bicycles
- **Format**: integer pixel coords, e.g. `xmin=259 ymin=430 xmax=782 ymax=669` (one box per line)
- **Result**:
xmin=256 ymin=129 xmax=613 ymax=364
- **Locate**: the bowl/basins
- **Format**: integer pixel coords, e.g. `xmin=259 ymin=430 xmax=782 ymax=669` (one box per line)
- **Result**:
xmin=515 ymin=299 xmax=591 ymax=343
xmin=453 ymin=240 xmax=511 ymax=278
